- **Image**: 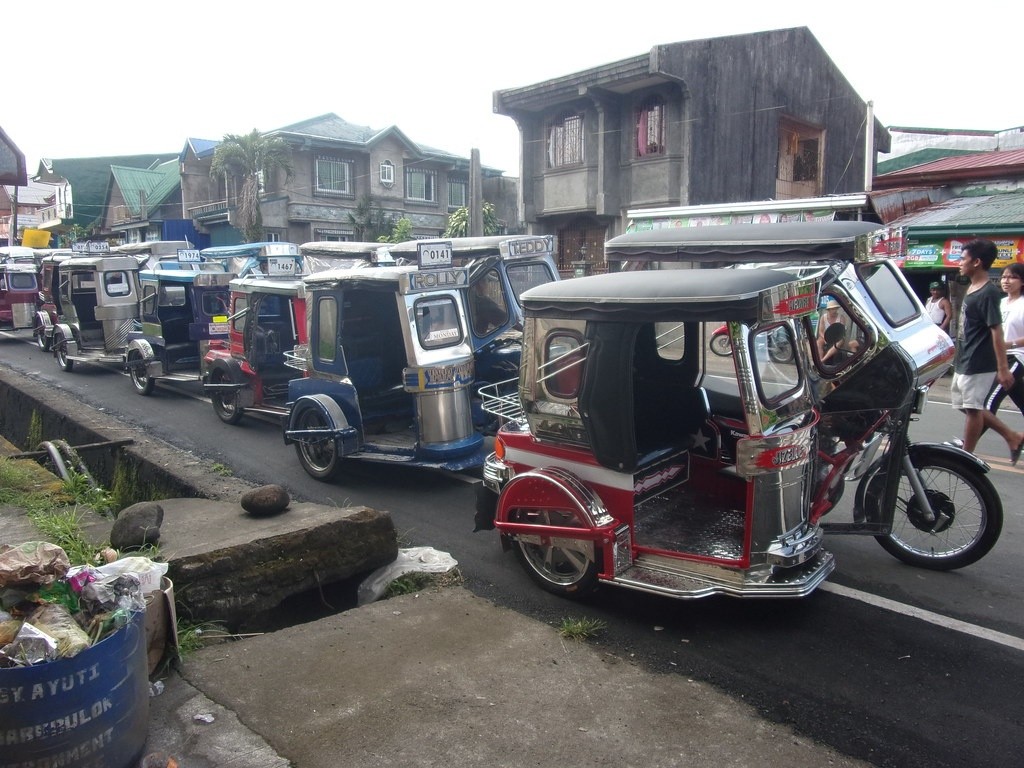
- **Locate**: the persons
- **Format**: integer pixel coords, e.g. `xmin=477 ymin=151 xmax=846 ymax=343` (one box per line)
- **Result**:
xmin=945 ymin=239 xmax=1024 ymax=468
xmin=816 ymin=301 xmax=865 ymax=368
xmin=447 ymin=276 xmax=524 ymax=335
xmin=924 ymin=282 xmax=952 ymax=331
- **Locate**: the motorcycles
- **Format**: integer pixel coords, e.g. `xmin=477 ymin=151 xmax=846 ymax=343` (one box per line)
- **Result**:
xmin=478 ymin=222 xmax=1005 ymax=601
xmin=0 ymin=233 xmax=585 ymax=483
xmin=709 ymin=323 xmax=795 ymax=365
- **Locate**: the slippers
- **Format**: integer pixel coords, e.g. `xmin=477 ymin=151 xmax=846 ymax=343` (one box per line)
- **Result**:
xmin=1010 ymin=437 xmax=1024 ymax=467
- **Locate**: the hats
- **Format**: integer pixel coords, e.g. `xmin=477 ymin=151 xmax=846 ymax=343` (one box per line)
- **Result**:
xmin=930 ymin=282 xmax=941 ymax=289
xmin=825 ymin=300 xmax=840 ymax=310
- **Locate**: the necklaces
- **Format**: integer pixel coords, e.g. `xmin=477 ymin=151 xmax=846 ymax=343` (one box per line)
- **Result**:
xmin=929 ymin=296 xmax=941 ymax=314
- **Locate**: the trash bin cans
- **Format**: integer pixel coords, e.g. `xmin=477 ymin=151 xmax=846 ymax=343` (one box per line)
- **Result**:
xmin=1 ymin=605 xmax=152 ymax=768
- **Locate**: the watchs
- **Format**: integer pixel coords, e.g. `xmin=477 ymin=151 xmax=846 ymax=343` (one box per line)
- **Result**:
xmin=1011 ymin=340 xmax=1017 ymax=348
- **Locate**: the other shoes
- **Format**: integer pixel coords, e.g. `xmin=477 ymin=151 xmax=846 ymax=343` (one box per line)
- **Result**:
xmin=942 ymin=439 xmax=965 ymax=448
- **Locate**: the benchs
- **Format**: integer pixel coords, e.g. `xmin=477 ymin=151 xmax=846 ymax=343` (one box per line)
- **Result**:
xmin=75 ymin=306 xmax=102 ymax=328
xmin=257 ymin=359 xmax=301 ymax=381
xmin=161 ymin=315 xmax=200 ymax=355
xmin=548 ymin=419 xmax=687 ymax=507
xmin=347 ymin=358 xmax=413 ymax=417
